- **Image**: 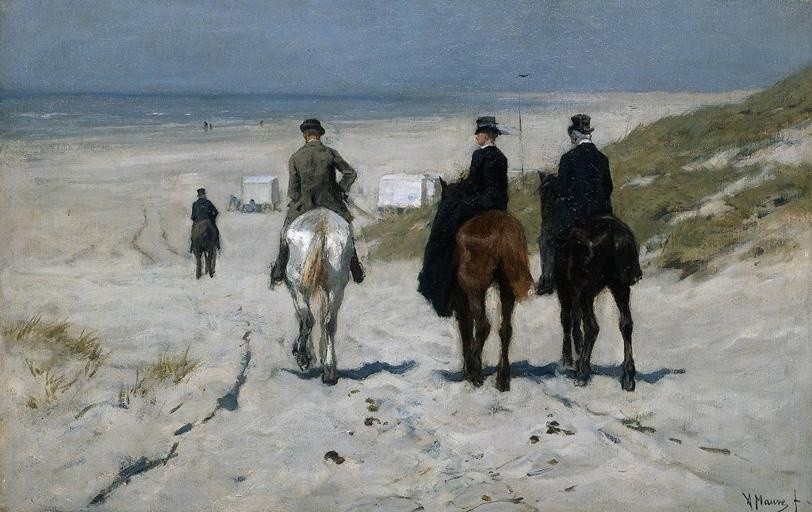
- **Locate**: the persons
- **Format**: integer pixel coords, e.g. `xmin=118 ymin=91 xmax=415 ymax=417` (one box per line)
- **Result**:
xmin=271 ymin=118 xmax=364 ymax=282
xmin=447 ymin=115 xmax=511 ymax=235
xmin=186 ymin=188 xmax=218 ymax=222
xmin=534 ymin=114 xmax=614 ymax=293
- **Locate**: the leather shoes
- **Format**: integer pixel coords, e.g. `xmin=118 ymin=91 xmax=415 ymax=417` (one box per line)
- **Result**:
xmin=534 ymin=286 xmax=553 ymax=296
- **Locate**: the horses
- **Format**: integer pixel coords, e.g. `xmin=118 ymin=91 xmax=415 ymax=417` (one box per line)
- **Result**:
xmin=284 ymin=206 xmax=354 ymax=384
xmin=439 ymin=174 xmax=537 ymax=391
xmin=192 ymin=220 xmax=217 ymax=280
xmin=538 ymin=170 xmax=643 ymax=391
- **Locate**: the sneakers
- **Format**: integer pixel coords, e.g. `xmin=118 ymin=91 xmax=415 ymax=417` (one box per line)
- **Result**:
xmin=270 ymin=256 xmax=284 ymax=281
xmin=349 ymin=257 xmax=364 ymax=283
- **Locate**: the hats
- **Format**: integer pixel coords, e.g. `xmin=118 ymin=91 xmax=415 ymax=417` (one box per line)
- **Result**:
xmin=300 ymin=119 xmax=325 ymax=136
xmin=569 ymin=114 xmax=595 ymax=134
xmin=475 ymin=116 xmax=501 ymax=136
